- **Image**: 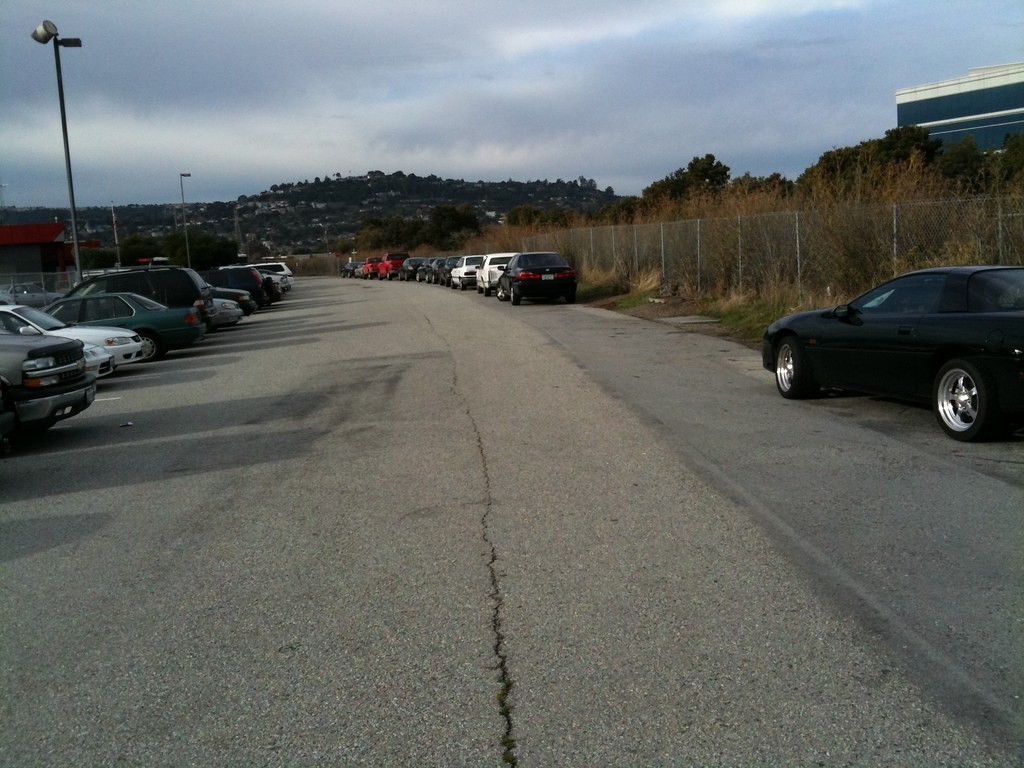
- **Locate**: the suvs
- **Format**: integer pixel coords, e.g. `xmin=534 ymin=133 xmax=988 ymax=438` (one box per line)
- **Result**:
xmin=64 ymin=265 xmax=220 ymax=345
xmin=0 ymin=334 xmax=99 ymax=451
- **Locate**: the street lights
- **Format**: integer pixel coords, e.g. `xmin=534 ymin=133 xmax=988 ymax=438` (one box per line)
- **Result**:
xmin=31 ymin=19 xmax=85 ymax=282
xmin=179 ymin=172 xmax=192 ymax=267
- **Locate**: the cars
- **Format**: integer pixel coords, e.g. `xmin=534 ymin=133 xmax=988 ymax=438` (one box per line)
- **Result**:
xmin=42 ymin=291 xmax=209 ymax=363
xmin=1 ymin=309 xmax=119 ymax=379
xmin=449 ymin=254 xmax=485 ymax=290
xmin=495 ymin=252 xmax=577 ymax=306
xmin=0 ymin=303 xmax=147 ymax=370
xmin=0 ymin=282 xmax=68 ymax=309
xmin=475 ymin=253 xmax=520 ymax=297
xmin=197 ymin=251 xmax=462 ymax=326
xmin=761 ymin=265 xmax=1024 ymax=442
